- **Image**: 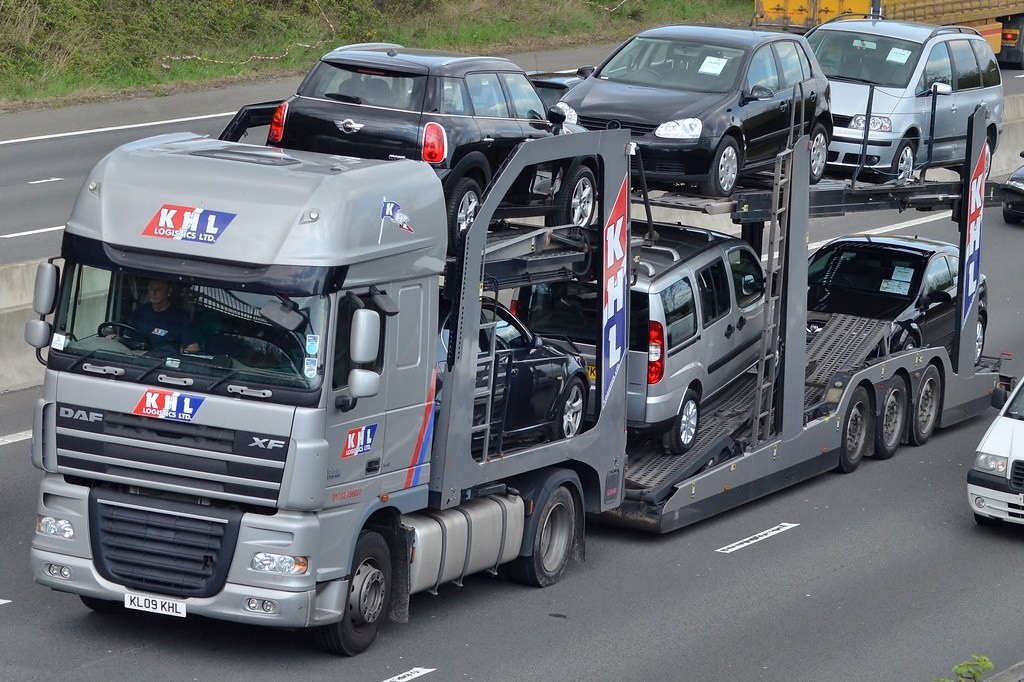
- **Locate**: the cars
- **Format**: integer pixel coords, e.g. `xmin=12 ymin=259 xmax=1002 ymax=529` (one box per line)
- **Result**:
xmin=966 ymin=374 xmax=1023 ymax=538
xmin=804 ymin=17 xmax=1002 ymax=183
xmin=433 ymin=291 xmax=590 ymax=456
xmin=557 ymin=26 xmax=836 ymax=197
xmin=786 ymin=233 xmax=990 ymax=368
xmin=1004 ymin=148 xmax=1023 ymax=228
xmin=270 ymin=43 xmax=602 ymax=241
xmin=511 ymin=221 xmax=771 ymax=455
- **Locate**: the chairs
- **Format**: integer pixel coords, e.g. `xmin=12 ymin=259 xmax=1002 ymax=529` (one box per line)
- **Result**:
xmin=339 ymin=77 xmax=365 ymax=102
xmin=825 ymin=38 xmax=850 ymax=76
xmin=460 ymin=76 xmax=495 ymax=116
xmin=366 ymin=78 xmax=391 ymax=100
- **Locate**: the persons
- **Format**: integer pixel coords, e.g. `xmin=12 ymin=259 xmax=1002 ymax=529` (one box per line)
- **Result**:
xmin=104 ymin=277 xmax=201 ymax=354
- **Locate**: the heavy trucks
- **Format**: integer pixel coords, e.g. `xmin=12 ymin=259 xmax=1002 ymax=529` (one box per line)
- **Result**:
xmin=754 ymin=0 xmax=1024 ymax=67
xmin=22 ymin=97 xmax=1023 ymax=650
